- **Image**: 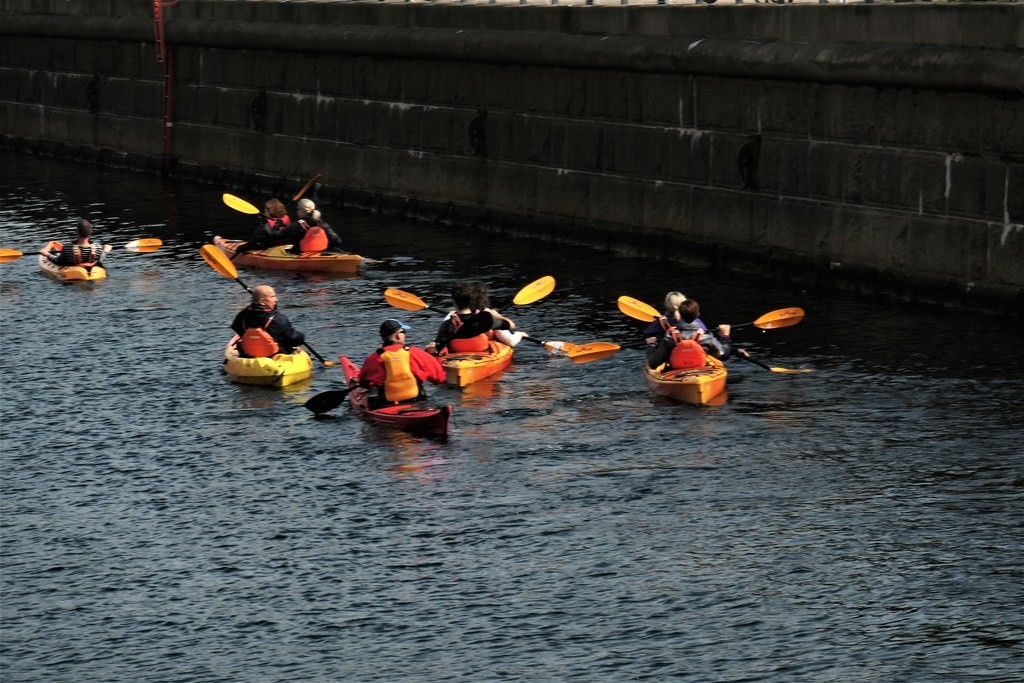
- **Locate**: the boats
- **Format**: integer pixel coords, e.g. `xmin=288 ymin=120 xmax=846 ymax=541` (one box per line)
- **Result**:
xmin=38 ymin=240 xmax=107 ymax=282
xmin=422 ymin=339 xmax=516 ymax=388
xmin=643 ymin=351 xmax=728 ymax=404
xmin=337 ymin=353 xmax=453 ymax=445
xmin=222 ymin=332 xmax=313 ymax=389
xmin=213 ymin=235 xmax=364 ymax=274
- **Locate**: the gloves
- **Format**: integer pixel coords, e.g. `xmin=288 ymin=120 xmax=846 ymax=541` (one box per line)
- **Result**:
xmin=236 ymin=243 xmax=252 ymax=253
xmin=274 ymin=219 xmax=283 ymax=227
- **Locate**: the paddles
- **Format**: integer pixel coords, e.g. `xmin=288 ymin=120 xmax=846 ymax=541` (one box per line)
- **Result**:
xmin=618 ymin=294 xmax=810 ymax=373
xmin=222 ymin=192 xmax=350 ymax=255
xmin=383 ymin=287 xmax=574 ymax=355
xmin=496 ymin=275 xmax=557 ymax=311
xmin=302 ymin=310 xmax=494 ymax=416
xmin=197 ymin=242 xmax=335 ymax=366
xmin=568 ymin=307 xmax=805 ymax=365
xmin=229 ymin=173 xmax=323 ymax=259
xmin=0 ymin=238 xmax=163 ymax=263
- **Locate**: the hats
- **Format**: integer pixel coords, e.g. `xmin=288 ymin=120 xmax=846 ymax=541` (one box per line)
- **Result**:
xmin=380 ymin=319 xmax=411 ymax=337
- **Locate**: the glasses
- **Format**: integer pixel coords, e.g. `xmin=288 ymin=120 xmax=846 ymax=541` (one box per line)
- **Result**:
xmin=396 ymin=330 xmax=406 ymax=334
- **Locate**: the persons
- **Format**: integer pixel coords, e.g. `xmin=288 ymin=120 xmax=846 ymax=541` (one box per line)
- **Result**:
xmin=231 ymin=285 xmax=305 ymax=351
xmin=39 ymin=220 xmax=103 ymax=267
xmin=247 ymin=198 xmax=342 ymax=250
xmin=644 ymin=291 xmax=750 ymax=373
xmin=358 ymin=320 xmax=447 ymax=404
xmin=436 ymin=280 xmax=529 ymax=352
